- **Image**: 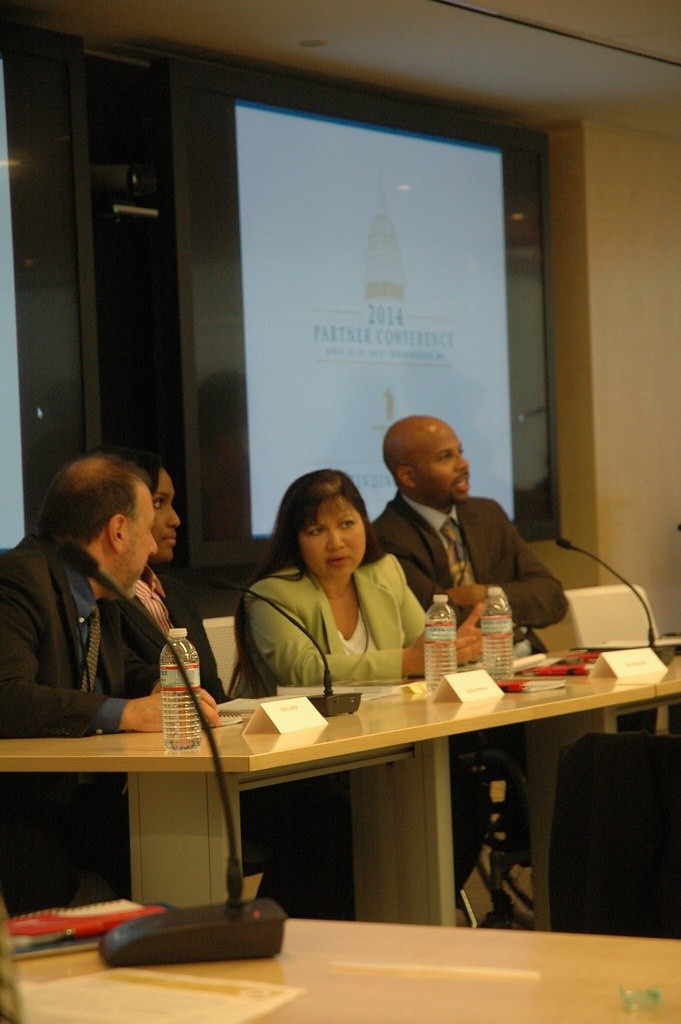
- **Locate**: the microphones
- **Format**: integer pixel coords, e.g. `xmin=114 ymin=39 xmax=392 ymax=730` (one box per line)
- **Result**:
xmin=209 ymin=577 xmax=363 ymax=717
xmin=556 ymin=538 xmax=673 ymax=663
xmin=51 ymin=541 xmax=285 ymax=966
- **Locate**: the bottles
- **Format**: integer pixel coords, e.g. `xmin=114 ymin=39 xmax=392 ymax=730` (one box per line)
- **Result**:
xmin=159 ymin=628 xmax=201 ymax=751
xmin=480 ymin=587 xmax=514 ymax=681
xmin=424 ymin=595 xmax=458 ymax=695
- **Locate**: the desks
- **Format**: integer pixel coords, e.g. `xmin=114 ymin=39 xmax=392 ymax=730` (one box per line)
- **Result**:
xmin=0 ymin=655 xmax=681 ymax=926
xmin=13 ymin=918 xmax=681 ymax=1024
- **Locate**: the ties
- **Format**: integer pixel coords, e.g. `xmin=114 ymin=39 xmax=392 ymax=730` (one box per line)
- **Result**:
xmin=443 ymin=519 xmax=474 ymax=588
xmin=79 ymin=602 xmax=103 ymax=697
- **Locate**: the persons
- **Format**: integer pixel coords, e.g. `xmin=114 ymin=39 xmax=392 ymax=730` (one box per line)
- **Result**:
xmin=0 ymin=450 xmax=353 ymax=920
xmin=371 ymin=415 xmax=589 ymax=928
xmin=229 ymin=468 xmax=492 ymax=921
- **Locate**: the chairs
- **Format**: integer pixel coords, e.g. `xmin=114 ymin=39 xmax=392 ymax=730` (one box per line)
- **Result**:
xmin=201 ymin=616 xmax=242 ymax=695
xmin=459 ymin=748 xmax=534 ymax=928
xmin=563 ymin=582 xmax=660 ymax=649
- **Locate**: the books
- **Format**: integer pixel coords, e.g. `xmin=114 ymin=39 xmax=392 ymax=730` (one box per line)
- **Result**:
xmin=0 ymin=899 xmax=179 ymax=959
xmin=519 ymin=650 xmax=599 ymax=675
xmin=497 ymin=678 xmax=565 ymax=693
